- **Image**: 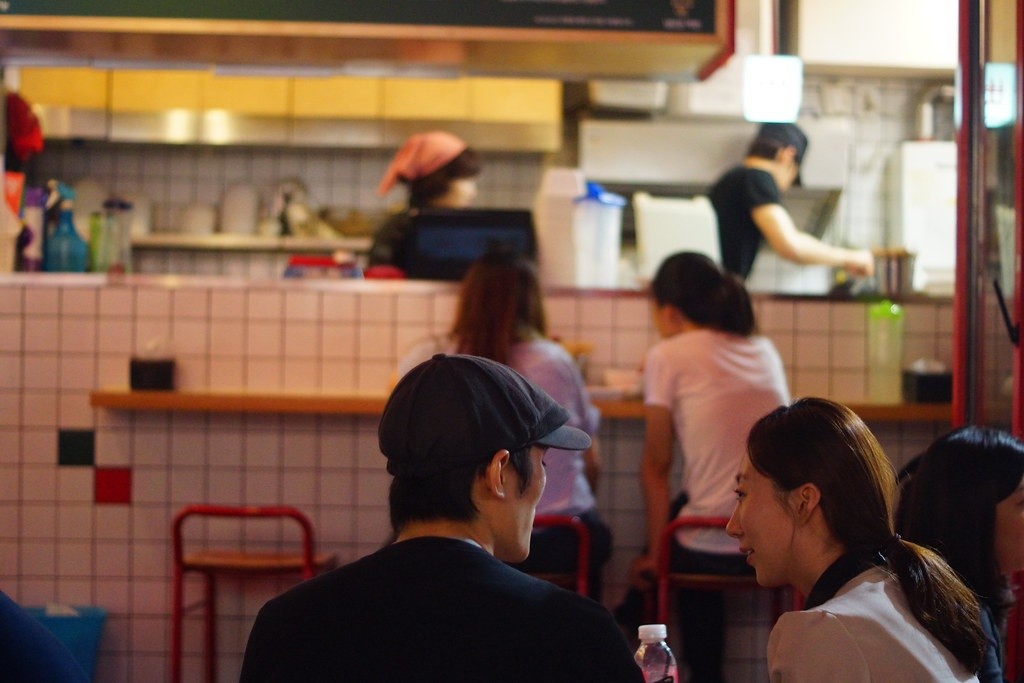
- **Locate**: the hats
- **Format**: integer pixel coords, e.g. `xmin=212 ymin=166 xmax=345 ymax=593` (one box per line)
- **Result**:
xmin=377 ymin=353 xmax=593 ymax=465
xmin=760 ymin=123 xmax=808 ymax=187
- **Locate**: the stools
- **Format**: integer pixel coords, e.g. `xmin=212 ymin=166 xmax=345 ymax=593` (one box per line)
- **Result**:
xmin=532 ymin=512 xmax=591 ymax=606
xmin=627 ymin=514 xmax=804 ymax=624
xmin=164 ymin=503 xmax=337 ymax=683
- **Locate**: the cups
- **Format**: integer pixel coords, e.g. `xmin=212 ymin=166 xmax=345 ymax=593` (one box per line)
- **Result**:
xmin=100 ymin=200 xmax=132 ymax=272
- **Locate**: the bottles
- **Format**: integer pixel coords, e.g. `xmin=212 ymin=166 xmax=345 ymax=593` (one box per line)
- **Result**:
xmin=867 ymin=300 xmax=903 ymax=403
xmin=573 ymin=180 xmax=626 ymax=287
xmin=634 ymin=624 xmax=677 ymax=683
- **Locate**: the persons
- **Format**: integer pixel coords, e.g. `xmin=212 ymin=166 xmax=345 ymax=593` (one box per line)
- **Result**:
xmin=726 ymin=397 xmax=980 ymax=683
xmin=891 ymin=424 xmax=1024 ymax=682
xmin=365 ymin=132 xmax=481 ymax=279
xmin=240 ymin=354 xmax=649 ymax=682
xmin=702 ymin=121 xmax=874 ymax=291
xmin=394 ymin=250 xmax=613 ymax=608
xmin=619 ymin=251 xmax=792 ymax=683
